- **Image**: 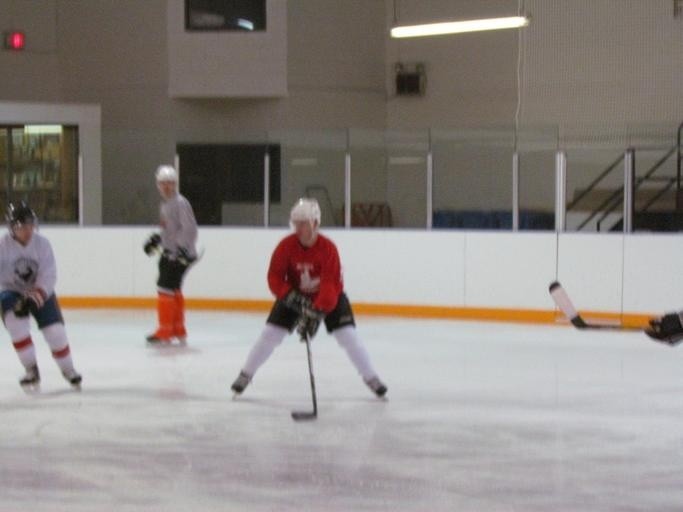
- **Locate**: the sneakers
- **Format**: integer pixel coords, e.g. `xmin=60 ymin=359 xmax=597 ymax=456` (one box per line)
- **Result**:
xmin=230 ymin=373 xmax=249 ymax=392
xmin=367 ymin=377 xmax=387 ymax=396
xmin=146 ymin=334 xmax=159 ymax=344
xmin=62 ymin=368 xmax=81 ymax=383
xmin=20 ymin=364 xmax=38 ymax=384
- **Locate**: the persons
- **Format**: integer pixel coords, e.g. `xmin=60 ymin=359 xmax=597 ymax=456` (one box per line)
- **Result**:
xmin=0 ymin=199 xmax=82 ymax=386
xmin=643 ymin=309 xmax=683 ymax=347
xmin=143 ymin=164 xmax=200 ymax=345
xmin=230 ymin=198 xmax=388 ymax=397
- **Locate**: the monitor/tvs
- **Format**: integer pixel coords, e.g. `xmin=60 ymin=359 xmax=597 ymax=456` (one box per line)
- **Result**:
xmin=184 ymin=0 xmax=267 ymax=32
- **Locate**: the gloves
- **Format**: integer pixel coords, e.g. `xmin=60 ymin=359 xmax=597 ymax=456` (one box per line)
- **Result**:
xmin=282 ymin=290 xmax=311 ymax=316
xmin=9 ymin=292 xmax=29 ymax=318
xmin=144 ymin=234 xmax=160 ymax=254
xmin=296 ymin=308 xmax=321 ymax=342
xmin=172 ymin=256 xmax=189 ymax=273
xmin=24 ymin=288 xmax=46 ymax=315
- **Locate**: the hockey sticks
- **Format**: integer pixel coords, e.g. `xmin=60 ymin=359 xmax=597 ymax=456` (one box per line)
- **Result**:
xmin=291 ymin=296 xmax=317 ymax=420
xmin=152 ymin=247 xmax=191 ymax=266
xmin=549 ymin=281 xmax=660 ymax=332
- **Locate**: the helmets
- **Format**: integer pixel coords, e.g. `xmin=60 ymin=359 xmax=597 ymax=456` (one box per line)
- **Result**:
xmin=155 ymin=165 xmax=177 ymax=188
xmin=289 ymin=198 xmax=321 ymax=234
xmin=4 ymin=199 xmax=39 ymax=234
